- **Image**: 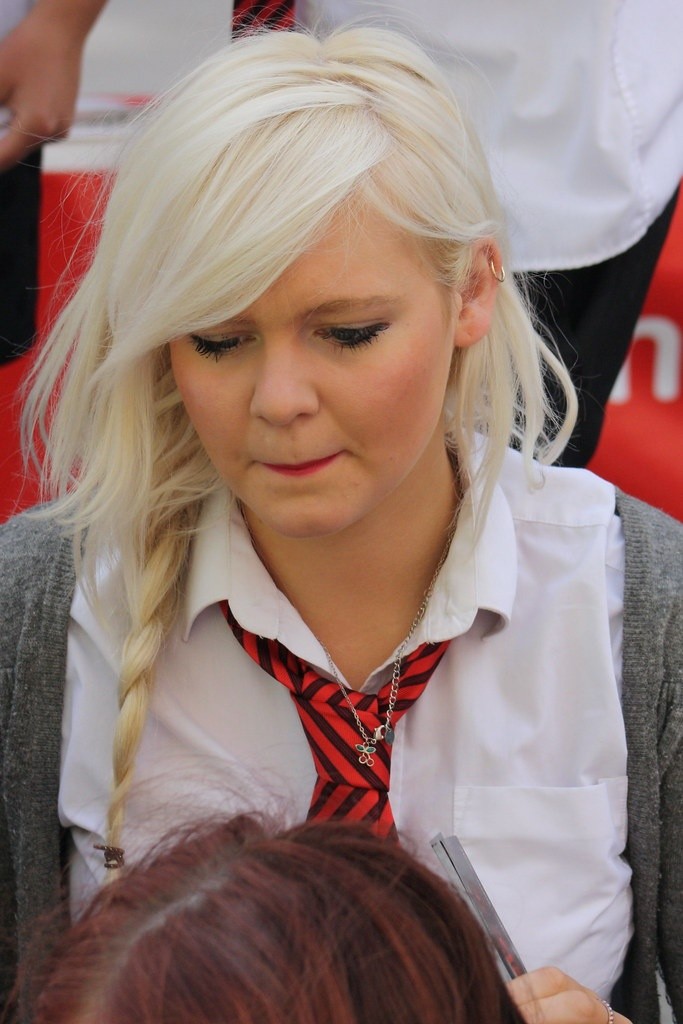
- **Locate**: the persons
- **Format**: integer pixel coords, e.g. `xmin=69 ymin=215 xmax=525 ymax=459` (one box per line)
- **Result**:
xmin=287 ymin=0 xmax=682 ymax=468
xmin=4 ymin=30 xmax=683 ymax=1024
xmin=0 ymin=1 xmax=109 ymax=368
xmin=0 ymin=800 xmax=532 ymax=1022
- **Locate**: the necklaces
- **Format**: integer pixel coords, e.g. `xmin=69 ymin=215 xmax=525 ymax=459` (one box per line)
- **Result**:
xmin=235 ymin=499 xmax=455 ymax=766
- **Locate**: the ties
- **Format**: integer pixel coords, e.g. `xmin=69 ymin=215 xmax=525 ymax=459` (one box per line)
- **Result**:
xmin=215 ymin=598 xmax=452 ymax=853
xmin=230 ymin=0 xmax=295 ymax=51
